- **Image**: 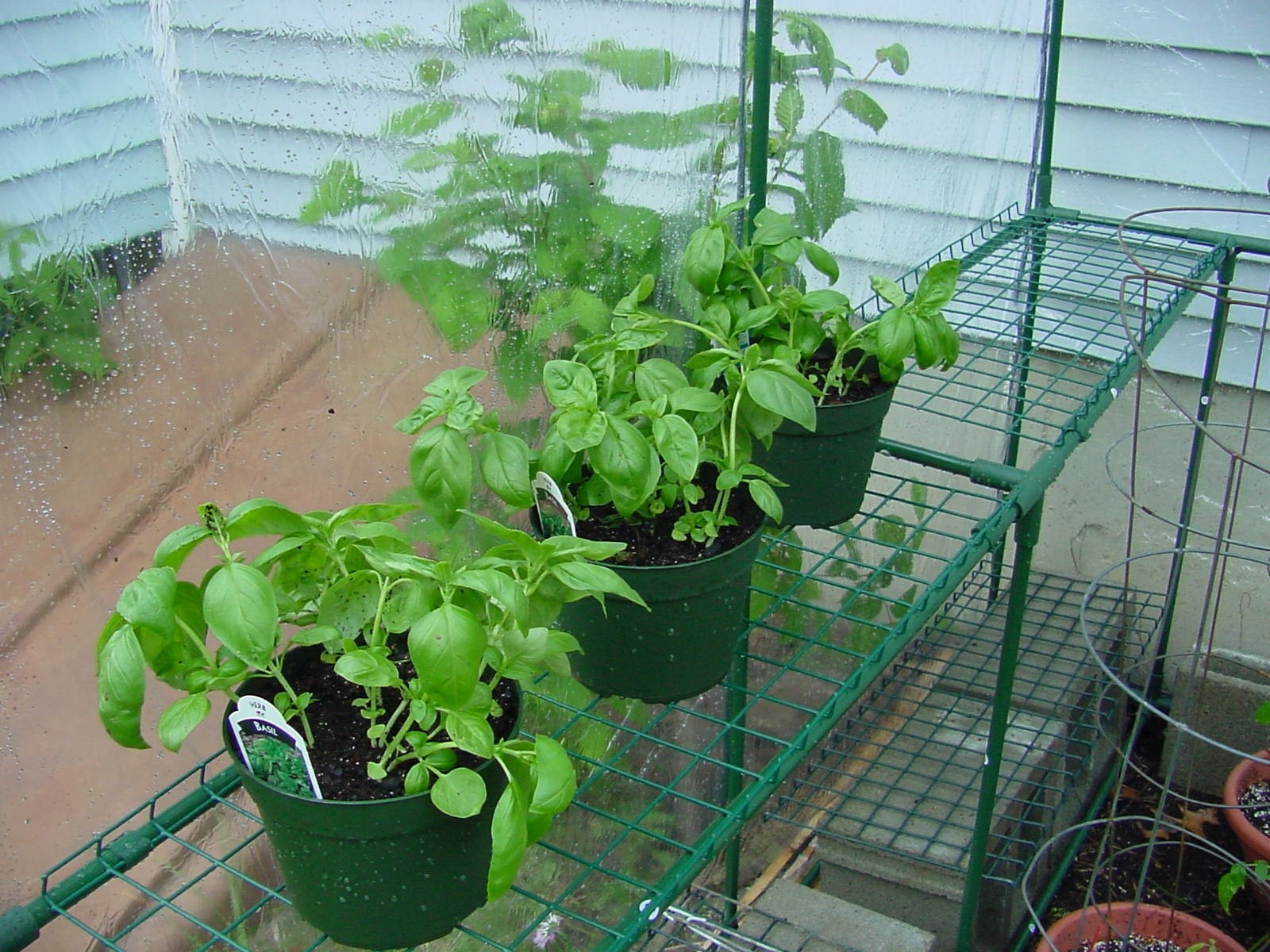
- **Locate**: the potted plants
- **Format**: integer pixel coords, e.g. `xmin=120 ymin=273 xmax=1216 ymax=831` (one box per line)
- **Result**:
xmin=96 ymin=367 xmax=650 ymax=952
xmin=1223 ymin=700 xmax=1270 ymax=910
xmin=410 ymin=279 xmax=831 ymax=707
xmin=684 ymin=187 xmax=959 ymax=528
xmin=1036 ymin=861 xmax=1270 ymax=952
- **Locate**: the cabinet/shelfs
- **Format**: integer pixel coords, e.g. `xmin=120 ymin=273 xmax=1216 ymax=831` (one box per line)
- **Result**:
xmin=0 ymin=208 xmax=1237 ymax=952
